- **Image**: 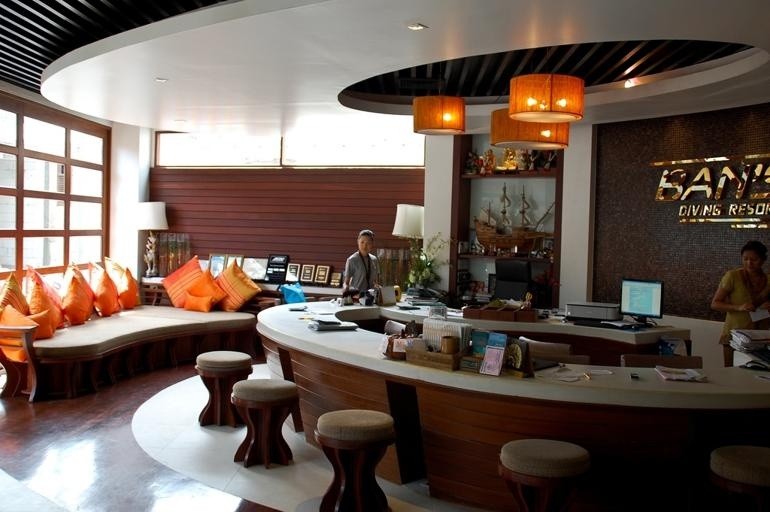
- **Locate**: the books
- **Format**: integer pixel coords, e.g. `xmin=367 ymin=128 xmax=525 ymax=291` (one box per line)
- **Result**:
xmin=654 ymin=364 xmax=712 ymax=383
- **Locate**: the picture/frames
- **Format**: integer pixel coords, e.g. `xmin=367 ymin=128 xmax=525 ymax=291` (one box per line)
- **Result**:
xmin=225 ymin=254 xmax=244 ymax=274
xmin=207 ymin=252 xmax=227 ymax=279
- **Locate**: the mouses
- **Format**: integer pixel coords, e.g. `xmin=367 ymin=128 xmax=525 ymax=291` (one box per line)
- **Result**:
xmin=632 ymin=325 xmax=639 ymax=331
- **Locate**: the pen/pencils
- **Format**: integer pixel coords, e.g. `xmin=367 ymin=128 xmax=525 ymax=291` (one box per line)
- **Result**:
xmin=583 ymin=373 xmax=590 ymax=380
xmin=758 ymin=376 xmax=770 ymax=380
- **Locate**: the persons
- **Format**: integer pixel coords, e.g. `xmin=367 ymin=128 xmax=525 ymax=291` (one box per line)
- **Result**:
xmin=710 ymin=240 xmax=770 ymax=367
xmin=342 ymin=229 xmax=383 ymax=294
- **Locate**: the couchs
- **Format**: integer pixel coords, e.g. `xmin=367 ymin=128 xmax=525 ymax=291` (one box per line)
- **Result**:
xmin=0 ymin=296 xmax=279 ymax=404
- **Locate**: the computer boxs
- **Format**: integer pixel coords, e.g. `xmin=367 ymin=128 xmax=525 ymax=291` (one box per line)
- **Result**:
xmin=566 ymin=303 xmax=621 ymax=320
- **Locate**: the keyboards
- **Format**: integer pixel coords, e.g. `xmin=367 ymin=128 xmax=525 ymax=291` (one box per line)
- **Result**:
xmin=574 ymin=321 xmax=633 ymax=330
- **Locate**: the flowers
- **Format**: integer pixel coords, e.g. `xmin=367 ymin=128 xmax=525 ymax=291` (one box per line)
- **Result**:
xmin=403 ymin=230 xmax=460 ymax=297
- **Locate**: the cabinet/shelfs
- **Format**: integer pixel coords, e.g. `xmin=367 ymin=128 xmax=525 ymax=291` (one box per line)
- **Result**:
xmin=448 ymin=135 xmax=565 ymax=310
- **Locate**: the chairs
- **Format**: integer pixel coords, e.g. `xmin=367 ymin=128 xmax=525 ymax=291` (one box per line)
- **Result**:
xmin=617 ymin=352 xmax=706 ymax=370
xmin=518 ymin=335 xmax=591 ymax=365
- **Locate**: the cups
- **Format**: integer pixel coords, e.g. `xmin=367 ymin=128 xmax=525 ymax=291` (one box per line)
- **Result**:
xmin=441 ymin=336 xmax=458 ymax=353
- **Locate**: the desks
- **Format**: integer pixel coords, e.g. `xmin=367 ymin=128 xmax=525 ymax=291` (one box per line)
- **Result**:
xmin=141 ymin=274 xmax=346 ymax=305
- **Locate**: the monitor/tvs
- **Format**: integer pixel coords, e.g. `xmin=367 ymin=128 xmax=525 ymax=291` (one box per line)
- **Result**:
xmin=619 ymin=278 xmax=664 ymax=328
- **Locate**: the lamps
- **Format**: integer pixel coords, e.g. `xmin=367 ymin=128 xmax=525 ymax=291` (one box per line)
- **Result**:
xmin=412 ymin=80 xmax=469 ymax=138
xmin=490 ymin=106 xmax=572 ymax=151
xmin=509 ymin=53 xmax=586 ymax=125
xmin=393 ymin=201 xmax=426 ymax=249
xmin=136 ymin=199 xmax=174 ymax=279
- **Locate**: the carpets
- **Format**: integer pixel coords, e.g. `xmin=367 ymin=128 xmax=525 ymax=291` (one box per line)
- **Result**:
xmin=129 ymin=362 xmax=489 ymax=511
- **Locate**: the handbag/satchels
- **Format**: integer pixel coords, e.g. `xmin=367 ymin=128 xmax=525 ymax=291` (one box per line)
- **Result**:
xmin=277 ymin=280 xmax=305 ymax=304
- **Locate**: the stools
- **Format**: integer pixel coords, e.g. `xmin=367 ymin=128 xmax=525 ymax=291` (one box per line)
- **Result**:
xmin=313 ymin=408 xmax=397 ymax=512
xmin=707 ymin=442 xmax=769 ymax=510
xmin=192 ymin=350 xmax=253 ymax=429
xmin=497 ymin=438 xmax=591 ymax=510
xmin=229 ymin=377 xmax=304 ymax=469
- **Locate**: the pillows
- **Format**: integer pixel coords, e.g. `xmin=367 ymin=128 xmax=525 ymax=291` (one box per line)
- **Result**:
xmin=0 ymin=253 xmax=264 ymax=338
